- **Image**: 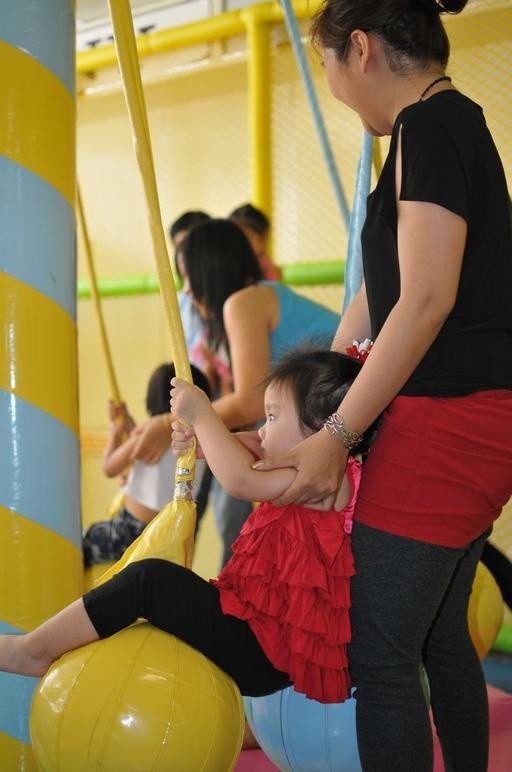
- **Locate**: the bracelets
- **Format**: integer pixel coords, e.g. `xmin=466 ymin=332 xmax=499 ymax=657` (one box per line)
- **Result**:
xmin=323 ymin=412 xmax=364 ymax=453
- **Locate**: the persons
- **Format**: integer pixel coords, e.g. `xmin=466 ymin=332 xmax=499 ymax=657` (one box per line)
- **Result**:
xmin=3 ymin=332 xmax=391 ymax=707
xmin=83 ymin=362 xmax=214 ymax=566
xmin=171 ymin=203 xmax=347 ymax=579
xmin=247 ymin=0 xmax=512 ymax=771
